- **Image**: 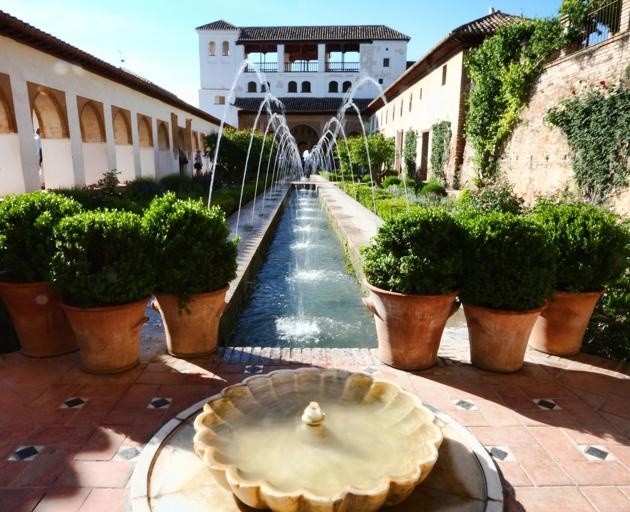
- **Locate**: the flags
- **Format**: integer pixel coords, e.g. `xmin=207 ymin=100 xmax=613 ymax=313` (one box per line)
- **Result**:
xmin=34 ymin=128 xmax=45 ymax=189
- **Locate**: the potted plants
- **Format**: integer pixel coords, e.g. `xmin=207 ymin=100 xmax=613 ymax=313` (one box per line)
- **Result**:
xmin=361 ymin=201 xmax=629 ymax=370
xmin=2 ymin=192 xmax=236 ymax=373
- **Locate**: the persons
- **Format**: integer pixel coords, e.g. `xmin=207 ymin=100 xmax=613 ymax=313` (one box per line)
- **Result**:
xmin=194 ymin=150 xmax=203 ymax=177
xmin=179 ymin=144 xmax=189 ymax=175
